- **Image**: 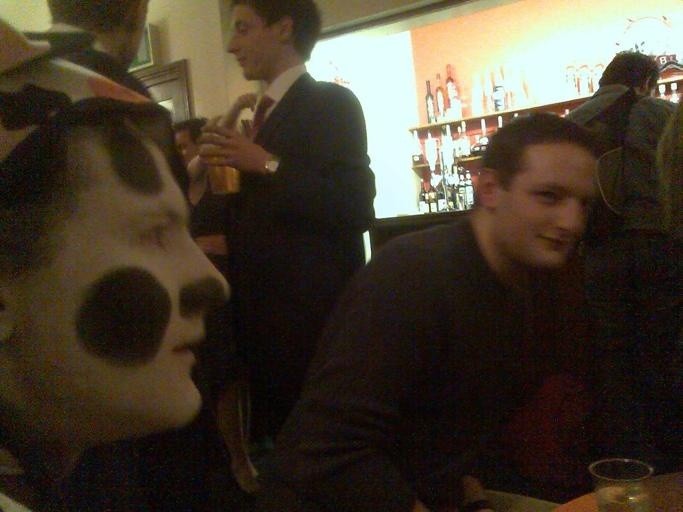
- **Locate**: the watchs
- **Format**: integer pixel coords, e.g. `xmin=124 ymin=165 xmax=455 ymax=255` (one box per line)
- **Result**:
xmin=263 ymin=154 xmax=279 ymax=177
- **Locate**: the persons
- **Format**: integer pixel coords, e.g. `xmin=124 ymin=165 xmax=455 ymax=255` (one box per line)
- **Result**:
xmin=260 ymin=115 xmax=642 ymax=509
xmin=565 ymin=50 xmax=683 ymax=475
xmin=1 ymin=0 xmax=258 ymax=283
xmin=198 ymin=1 xmax=376 ymax=447
xmin=0 ymin=18 xmax=270 ymax=511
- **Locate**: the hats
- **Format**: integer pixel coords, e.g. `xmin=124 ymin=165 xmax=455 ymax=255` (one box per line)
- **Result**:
xmin=0 ymin=16 xmax=169 ymax=170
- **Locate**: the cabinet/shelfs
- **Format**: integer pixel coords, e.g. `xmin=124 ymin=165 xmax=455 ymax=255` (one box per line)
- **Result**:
xmin=409 ymin=58 xmax=683 ymax=209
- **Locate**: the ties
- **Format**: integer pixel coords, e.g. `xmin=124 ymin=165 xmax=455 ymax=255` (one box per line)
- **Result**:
xmin=252 ymin=97 xmax=273 ymax=139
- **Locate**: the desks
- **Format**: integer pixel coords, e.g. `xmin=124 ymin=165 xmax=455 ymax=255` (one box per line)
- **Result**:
xmin=369 ymin=215 xmax=458 ymax=261
xmin=550 ymin=473 xmax=682 ymax=511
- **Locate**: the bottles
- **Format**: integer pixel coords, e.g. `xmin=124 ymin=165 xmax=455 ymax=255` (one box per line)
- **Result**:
xmin=418 ymin=64 xmax=515 ymax=215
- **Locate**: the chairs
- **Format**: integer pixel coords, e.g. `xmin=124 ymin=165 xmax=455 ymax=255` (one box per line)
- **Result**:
xmin=216 ymin=366 xmax=305 ymax=494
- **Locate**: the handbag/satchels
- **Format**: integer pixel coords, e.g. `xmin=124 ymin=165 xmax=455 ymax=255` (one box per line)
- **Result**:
xmin=582 ymin=88 xmax=641 ymax=232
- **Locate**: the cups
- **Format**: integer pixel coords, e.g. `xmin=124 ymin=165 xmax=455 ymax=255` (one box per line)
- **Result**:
xmin=587 ymin=457 xmax=654 ymax=510
xmin=201 ymin=133 xmax=242 ymax=196
